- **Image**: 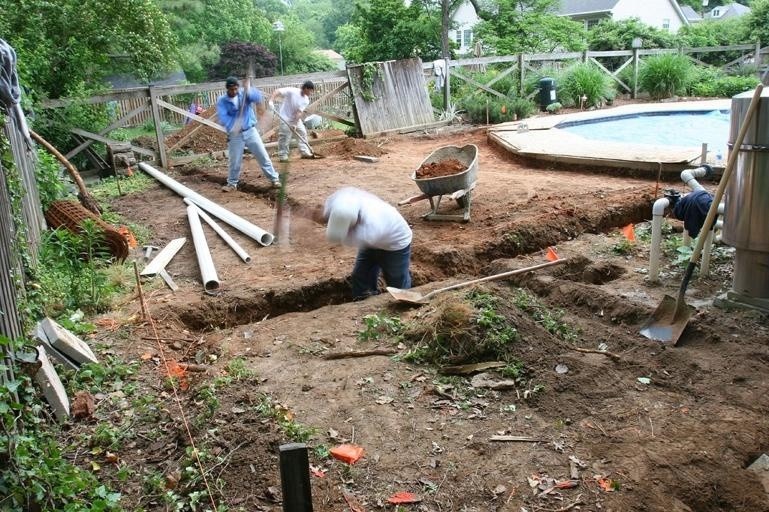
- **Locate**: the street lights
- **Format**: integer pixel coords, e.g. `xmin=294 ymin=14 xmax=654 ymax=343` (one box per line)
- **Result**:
xmin=272 ymin=21 xmax=286 ymax=77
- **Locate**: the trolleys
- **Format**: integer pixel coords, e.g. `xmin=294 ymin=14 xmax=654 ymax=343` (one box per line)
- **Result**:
xmin=397 ymin=142 xmax=480 ymax=223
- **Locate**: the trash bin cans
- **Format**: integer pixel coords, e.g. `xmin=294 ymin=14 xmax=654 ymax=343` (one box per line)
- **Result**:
xmin=540 ymin=78 xmax=558 ymax=112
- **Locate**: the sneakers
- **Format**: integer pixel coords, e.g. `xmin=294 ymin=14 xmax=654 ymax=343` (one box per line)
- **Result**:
xmin=221 ymin=184 xmax=237 ymax=192
xmin=302 ymin=154 xmax=313 ymax=158
xmin=272 ymin=182 xmax=282 ymax=188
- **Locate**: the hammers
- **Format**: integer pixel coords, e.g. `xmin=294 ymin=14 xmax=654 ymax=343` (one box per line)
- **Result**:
xmin=143 ymin=245 xmax=159 ymax=261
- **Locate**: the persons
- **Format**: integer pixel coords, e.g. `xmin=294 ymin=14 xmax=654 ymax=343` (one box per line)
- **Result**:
xmin=216 ymin=76 xmax=282 ymax=192
xmin=322 ymin=187 xmax=413 ymax=301
xmin=268 ymin=81 xmax=315 ymax=162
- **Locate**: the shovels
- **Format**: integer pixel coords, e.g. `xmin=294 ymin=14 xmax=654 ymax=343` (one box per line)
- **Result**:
xmin=386 ymin=257 xmax=567 ymax=304
xmin=639 ymin=82 xmax=764 ymax=348
xmin=275 ymin=110 xmax=323 ymax=156
